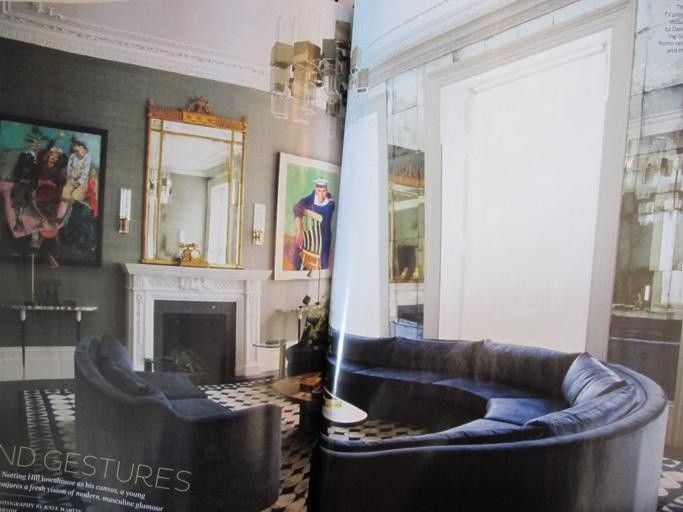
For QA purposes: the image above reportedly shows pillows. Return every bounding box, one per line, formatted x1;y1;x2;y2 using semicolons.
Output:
100;332;170;406
320;330;638;450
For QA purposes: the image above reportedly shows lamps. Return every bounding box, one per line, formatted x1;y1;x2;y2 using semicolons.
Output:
252;203;266;246
117;187;132;234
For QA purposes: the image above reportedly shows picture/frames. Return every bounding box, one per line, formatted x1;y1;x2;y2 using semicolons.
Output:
272;152;339;281
0;113;108;266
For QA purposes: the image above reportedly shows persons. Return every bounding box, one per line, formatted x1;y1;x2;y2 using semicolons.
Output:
9;139;97;236
283;176;334;271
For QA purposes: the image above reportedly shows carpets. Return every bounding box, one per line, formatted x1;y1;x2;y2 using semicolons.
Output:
18;371;683;512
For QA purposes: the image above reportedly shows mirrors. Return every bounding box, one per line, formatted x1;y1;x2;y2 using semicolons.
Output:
386;144;426;283
140;99;248;269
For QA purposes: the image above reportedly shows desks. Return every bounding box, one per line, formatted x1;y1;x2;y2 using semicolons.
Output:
277;302;324;344
273;372;319;440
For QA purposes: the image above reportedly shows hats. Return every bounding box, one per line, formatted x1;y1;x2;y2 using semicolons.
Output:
313;177;330;188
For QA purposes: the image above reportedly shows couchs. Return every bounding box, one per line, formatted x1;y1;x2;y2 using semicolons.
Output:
74;331;282;512
307;329;669;512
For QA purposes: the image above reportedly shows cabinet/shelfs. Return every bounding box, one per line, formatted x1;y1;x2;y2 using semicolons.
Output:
611;315;682;463
